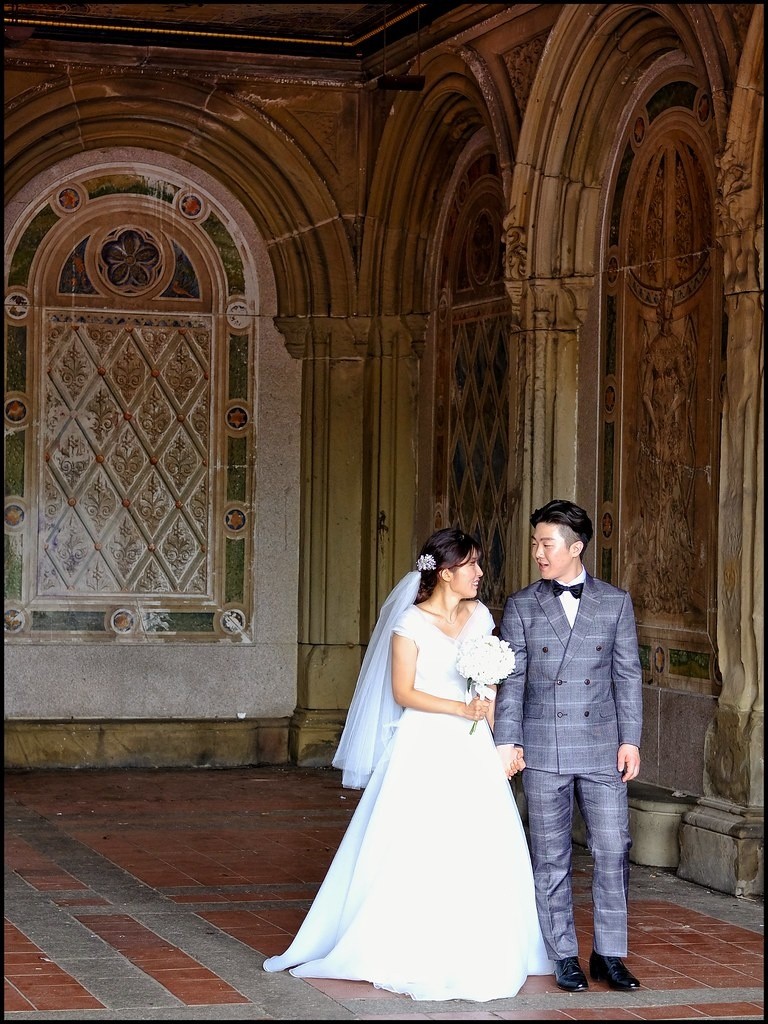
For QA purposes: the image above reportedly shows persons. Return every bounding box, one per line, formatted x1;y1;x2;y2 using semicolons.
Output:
264;528;554;1002
494;500;643;994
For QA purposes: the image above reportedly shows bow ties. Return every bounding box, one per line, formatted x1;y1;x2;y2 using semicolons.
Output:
551;579;584;599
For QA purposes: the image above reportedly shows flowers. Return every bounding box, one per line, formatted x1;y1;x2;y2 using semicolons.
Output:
454;631;515;735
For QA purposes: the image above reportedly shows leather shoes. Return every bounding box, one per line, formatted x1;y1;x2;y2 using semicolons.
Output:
590;951;639;991
556;957;588;992
